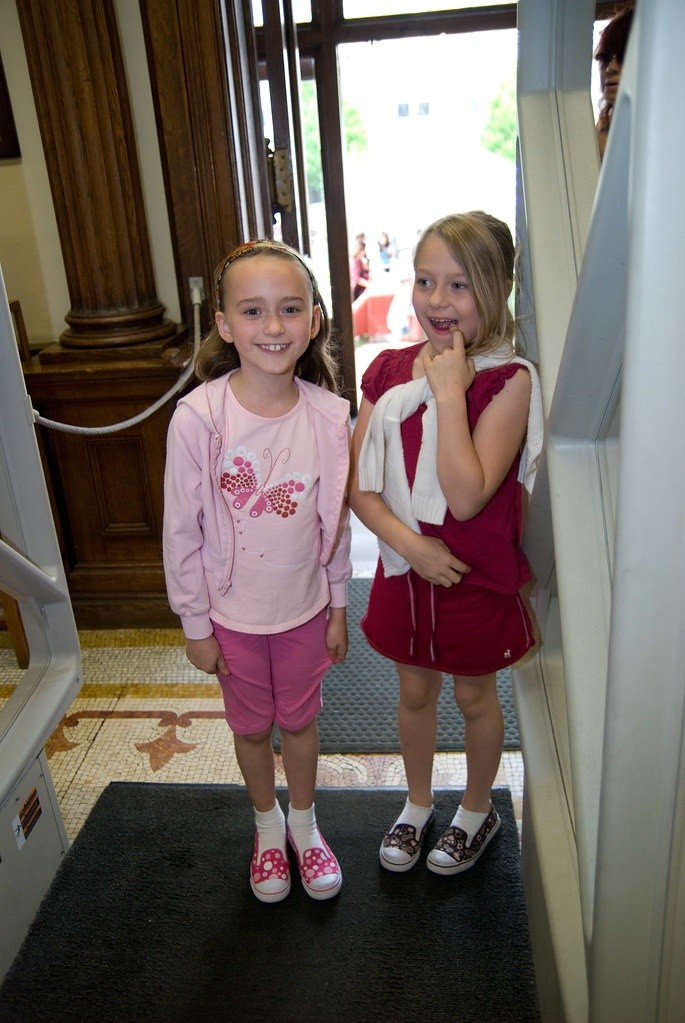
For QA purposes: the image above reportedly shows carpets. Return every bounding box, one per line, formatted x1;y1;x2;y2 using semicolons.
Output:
267;577;519;754
0;780;544;1023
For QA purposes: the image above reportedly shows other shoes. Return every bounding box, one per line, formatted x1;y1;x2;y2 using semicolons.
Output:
250;830;292;904
426;800;501;875
379;803;435;872
288;826;343;900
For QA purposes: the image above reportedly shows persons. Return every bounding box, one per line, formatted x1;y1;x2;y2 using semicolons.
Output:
348;211;545;877
353;230;423;341
162;239;353;902
596;8;634;160
